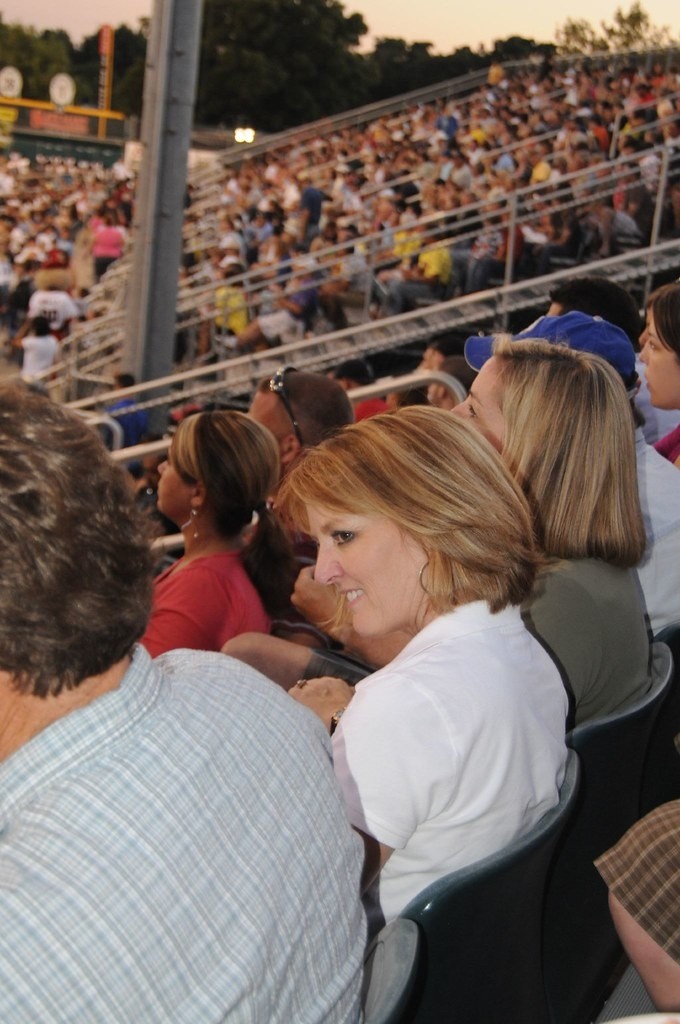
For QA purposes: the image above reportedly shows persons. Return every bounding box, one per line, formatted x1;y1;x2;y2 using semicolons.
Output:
0;43;680;728
595;800;679;1024
275;406;570;932
0;388;367;1024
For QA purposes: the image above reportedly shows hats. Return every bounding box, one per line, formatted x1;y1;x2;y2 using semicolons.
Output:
462;311;639;402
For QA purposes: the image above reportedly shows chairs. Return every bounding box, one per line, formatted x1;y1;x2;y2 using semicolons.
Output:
341;618;680;1024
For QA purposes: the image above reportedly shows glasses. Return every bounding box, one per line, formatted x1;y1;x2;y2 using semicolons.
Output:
269;365;306;447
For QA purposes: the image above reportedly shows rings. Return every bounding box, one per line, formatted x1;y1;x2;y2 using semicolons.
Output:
296;679;307;688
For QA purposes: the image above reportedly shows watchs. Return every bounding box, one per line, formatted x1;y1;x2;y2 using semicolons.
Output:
330;706;347;735
